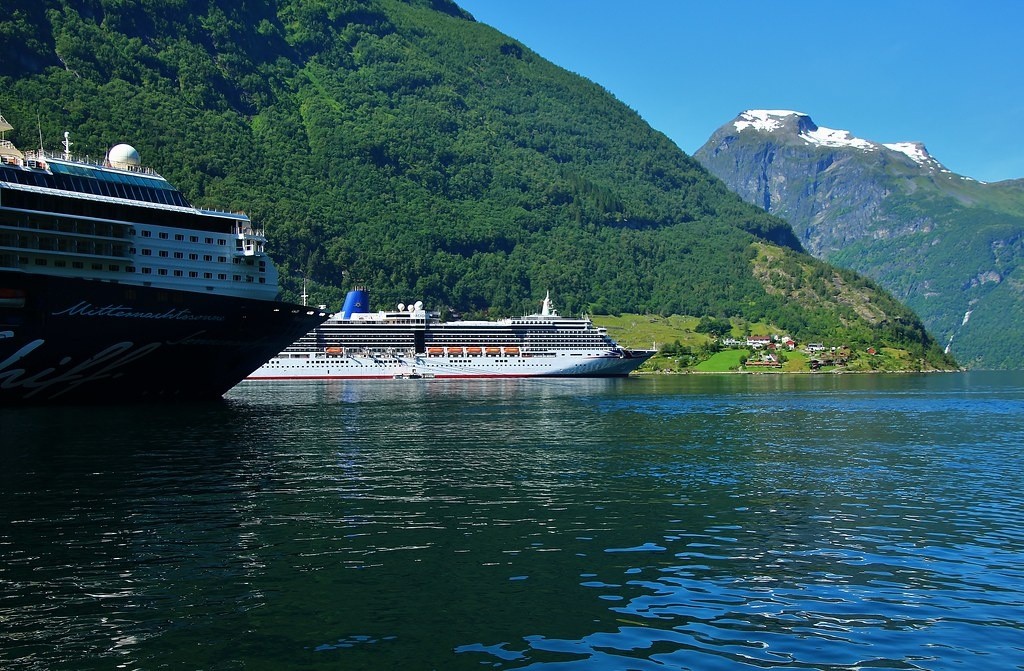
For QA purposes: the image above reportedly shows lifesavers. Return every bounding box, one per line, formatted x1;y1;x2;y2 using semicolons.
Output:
356;303;361;308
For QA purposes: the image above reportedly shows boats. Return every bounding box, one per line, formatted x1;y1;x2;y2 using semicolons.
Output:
466;348;482;357
326;348;343;357
0;108;337;411
504;347;519;356
447;348;464;356
428;348;444;356
486;348;501;356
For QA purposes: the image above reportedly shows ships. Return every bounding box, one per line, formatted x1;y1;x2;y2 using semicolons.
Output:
243;287;659;381
